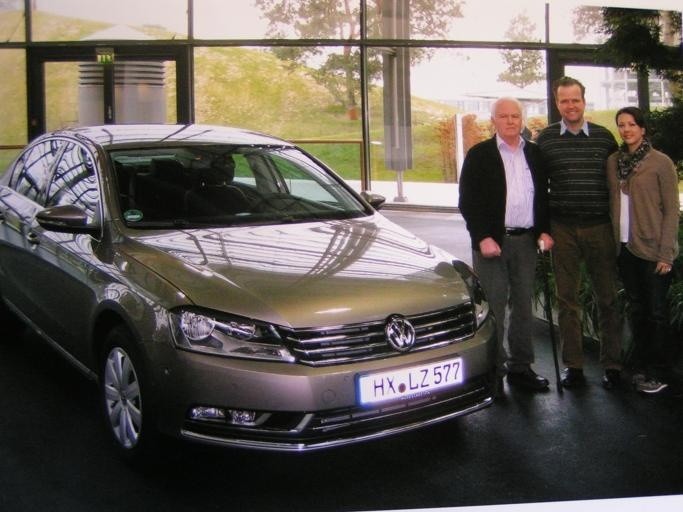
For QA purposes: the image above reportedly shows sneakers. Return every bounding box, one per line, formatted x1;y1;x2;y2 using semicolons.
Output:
632;374;668;394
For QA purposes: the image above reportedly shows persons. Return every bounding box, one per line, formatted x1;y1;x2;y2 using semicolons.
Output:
532;78;625;393
606;107;679;395
456;97;556;399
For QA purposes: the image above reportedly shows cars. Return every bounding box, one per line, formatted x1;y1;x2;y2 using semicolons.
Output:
0;124;496;459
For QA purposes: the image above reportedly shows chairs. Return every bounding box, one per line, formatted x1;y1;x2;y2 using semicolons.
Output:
115;151;252;217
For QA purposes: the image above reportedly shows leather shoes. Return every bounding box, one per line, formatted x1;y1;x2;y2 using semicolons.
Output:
507;368;549;387
560;367;583;386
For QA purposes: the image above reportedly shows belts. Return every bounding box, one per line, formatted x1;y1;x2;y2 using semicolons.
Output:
505;227;528;240
600;368;619;388
